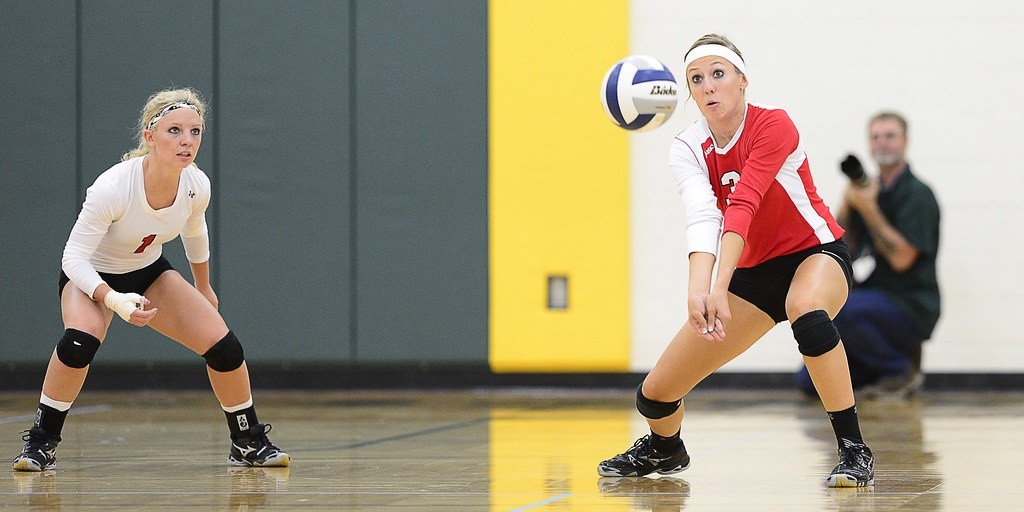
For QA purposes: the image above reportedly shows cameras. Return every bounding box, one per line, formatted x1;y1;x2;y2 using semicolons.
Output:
840;153;871;188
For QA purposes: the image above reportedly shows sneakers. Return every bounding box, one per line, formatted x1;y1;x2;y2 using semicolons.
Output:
597;432;691;478
826;437;875;487
13;420;62;472
227;423;290;469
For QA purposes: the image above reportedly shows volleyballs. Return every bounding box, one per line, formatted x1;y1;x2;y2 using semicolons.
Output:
602;54;680;134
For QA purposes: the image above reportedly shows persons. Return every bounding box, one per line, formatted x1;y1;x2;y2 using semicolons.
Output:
596;32;876;488
796;111;941;403
10;87;291;473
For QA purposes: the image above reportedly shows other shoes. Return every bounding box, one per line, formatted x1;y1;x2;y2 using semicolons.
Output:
862;368;926;401
